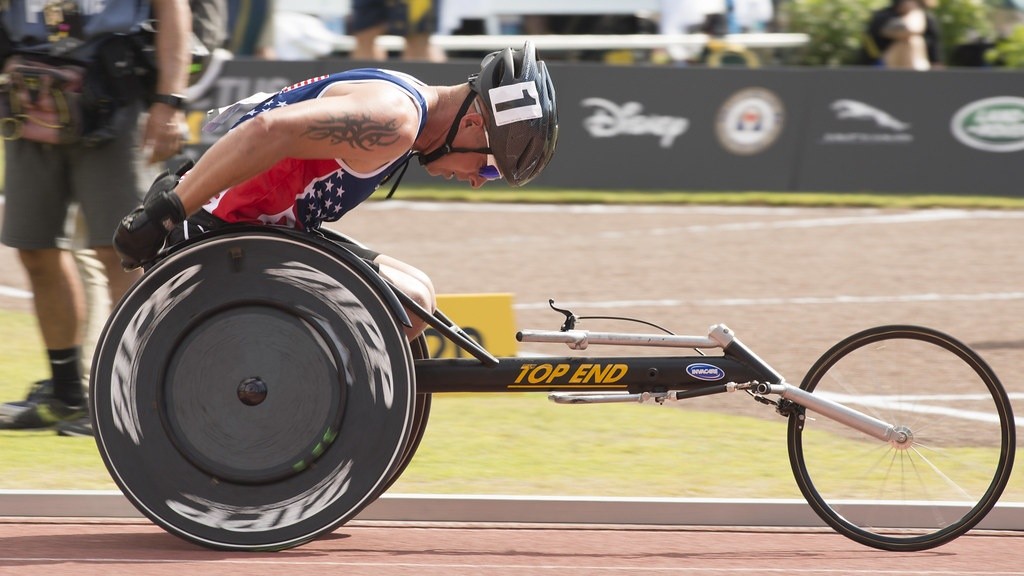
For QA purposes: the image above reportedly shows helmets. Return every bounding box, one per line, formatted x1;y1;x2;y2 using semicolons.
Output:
471;40;559;188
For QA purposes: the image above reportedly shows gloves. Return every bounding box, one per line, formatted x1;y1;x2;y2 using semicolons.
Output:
112;191;187;274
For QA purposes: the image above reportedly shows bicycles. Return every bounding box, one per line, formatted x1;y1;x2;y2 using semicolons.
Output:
89;221;1016;555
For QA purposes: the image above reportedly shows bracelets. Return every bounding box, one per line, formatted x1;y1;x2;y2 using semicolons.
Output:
154;94;192;110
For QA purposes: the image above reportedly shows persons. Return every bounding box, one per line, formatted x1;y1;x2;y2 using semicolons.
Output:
190;0;228;57
854;1;941;72
949;0;1024;68
686;14;762;68
603;17;672;65
114;42;558;343
343;1;447;63
1;0;192;436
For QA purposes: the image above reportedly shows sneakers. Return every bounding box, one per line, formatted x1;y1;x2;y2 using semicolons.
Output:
1;378;92;438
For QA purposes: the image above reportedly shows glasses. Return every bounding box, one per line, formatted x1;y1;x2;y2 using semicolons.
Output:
474;92;503;182
